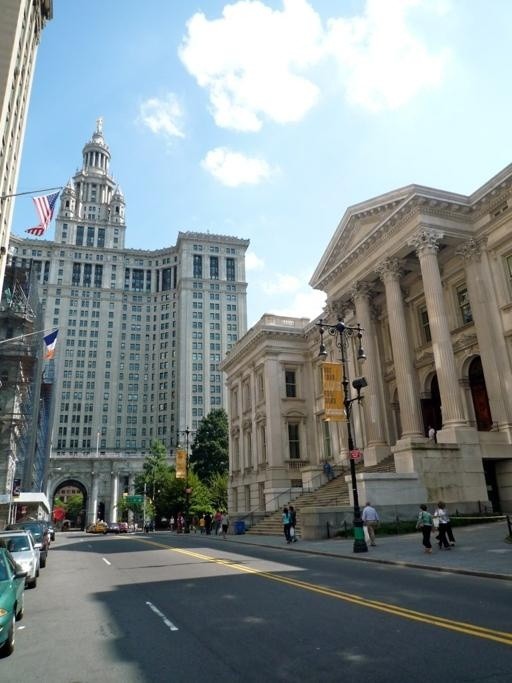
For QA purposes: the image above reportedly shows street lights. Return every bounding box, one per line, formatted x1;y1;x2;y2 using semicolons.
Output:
173;425;201;534
38;467;61;492
313;314;372;554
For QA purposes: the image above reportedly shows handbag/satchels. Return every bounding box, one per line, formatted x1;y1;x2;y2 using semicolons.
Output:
290;528;294;537
432;517;440;529
419;520;425;527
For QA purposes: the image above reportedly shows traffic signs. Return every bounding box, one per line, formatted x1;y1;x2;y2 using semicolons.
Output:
127;495;144;502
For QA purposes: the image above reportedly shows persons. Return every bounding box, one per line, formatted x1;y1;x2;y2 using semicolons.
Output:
280;507;293;544
287;505;297;542
144;520;153;537
433;500;451;550
323;459;337;481
168;508;223;536
435;505;456;547
219;510;229;539
361;501;380;546
415;503;435;553
427;424;436;440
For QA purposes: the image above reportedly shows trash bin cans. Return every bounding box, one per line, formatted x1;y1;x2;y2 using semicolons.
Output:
234;522;245;535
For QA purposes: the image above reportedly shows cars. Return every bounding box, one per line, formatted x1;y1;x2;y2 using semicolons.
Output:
0;517;56;655
87;518;130;534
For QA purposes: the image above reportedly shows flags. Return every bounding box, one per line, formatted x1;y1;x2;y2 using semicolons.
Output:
175;448;187;478
24;190;60;237
320;361;348;422
42;328;59;361
